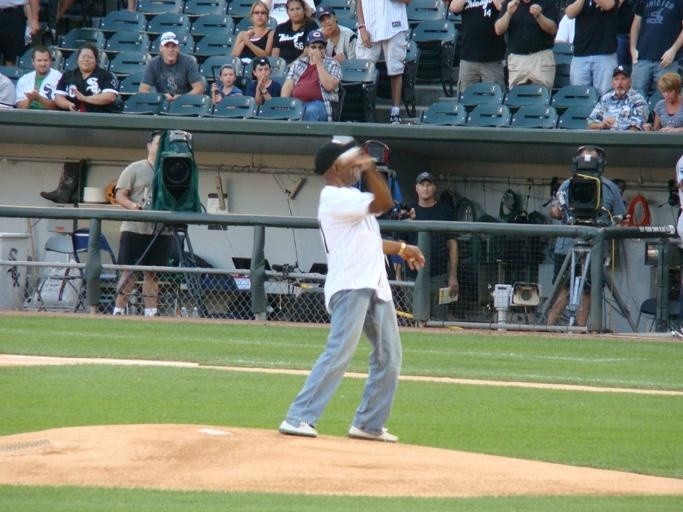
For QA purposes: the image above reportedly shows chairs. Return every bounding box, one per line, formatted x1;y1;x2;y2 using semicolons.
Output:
27;228;253;322
1;1;683;134
456;228;533;284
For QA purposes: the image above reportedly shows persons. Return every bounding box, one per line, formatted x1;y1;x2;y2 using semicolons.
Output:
278;133;402;443
112;133;166;315
545;145;631;327
673;155;683;336
392;172;462;322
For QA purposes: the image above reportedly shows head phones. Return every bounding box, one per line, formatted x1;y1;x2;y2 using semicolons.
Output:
574;145;610;175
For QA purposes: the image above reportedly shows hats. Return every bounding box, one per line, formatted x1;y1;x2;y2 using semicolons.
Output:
315;5;333;20
416;172;435;184
251;56;271;80
305;30;327;46
614;65;630;76
313;135;360;174
159;32;180;46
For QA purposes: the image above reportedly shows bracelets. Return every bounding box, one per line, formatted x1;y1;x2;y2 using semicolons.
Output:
398;243;406;257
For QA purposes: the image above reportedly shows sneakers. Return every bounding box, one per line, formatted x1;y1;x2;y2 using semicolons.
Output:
279;419;317;437
388;114;403;124
348;424;399;442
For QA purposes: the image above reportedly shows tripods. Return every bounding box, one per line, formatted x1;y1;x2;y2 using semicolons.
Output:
536;241;637;334
100;223;211;319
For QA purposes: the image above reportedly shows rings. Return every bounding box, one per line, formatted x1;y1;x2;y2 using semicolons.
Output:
410;258;416;262
